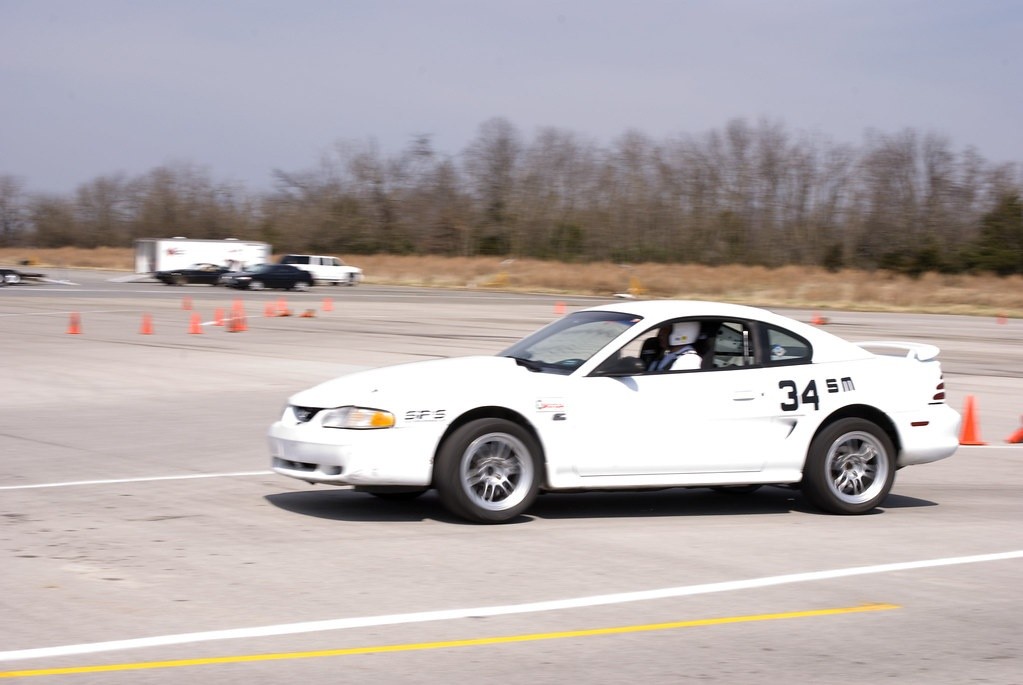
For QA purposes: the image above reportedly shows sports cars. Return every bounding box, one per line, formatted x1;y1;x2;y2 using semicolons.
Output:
270;298;964;522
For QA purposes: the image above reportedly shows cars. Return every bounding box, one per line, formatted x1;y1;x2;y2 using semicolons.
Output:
0;263;47;286
219;262;314;295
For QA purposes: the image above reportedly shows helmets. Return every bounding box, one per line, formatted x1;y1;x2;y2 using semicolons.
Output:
657;320;700;346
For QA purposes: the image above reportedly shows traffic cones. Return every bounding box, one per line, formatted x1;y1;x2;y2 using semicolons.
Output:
138;310;154;334
189;311;206;335
323;295;335;312
1007;412;1023;448
995;311;1006;329
182;294;195;311
211;305;225;327
806;310;828;326
66;309;83;333
295;308;316;319
225;290;249;332
267;298;291;318
555;301;566;316
958;394;988;447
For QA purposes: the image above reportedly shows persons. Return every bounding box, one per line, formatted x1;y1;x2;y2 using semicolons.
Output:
646;322;703;370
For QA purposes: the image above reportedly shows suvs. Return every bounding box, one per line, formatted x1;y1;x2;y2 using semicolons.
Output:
275;254;365;284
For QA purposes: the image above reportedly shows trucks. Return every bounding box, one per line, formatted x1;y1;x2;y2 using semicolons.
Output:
135;236;272;284
